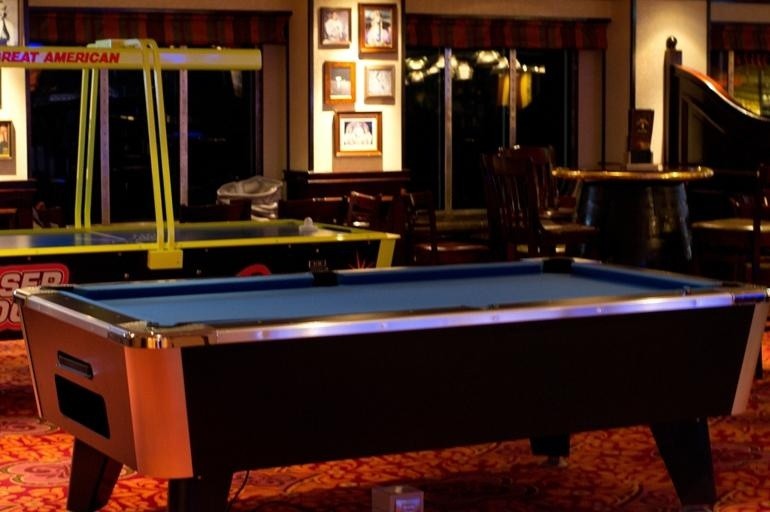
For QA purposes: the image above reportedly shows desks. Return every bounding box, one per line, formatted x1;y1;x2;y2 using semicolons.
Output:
9;255;770;511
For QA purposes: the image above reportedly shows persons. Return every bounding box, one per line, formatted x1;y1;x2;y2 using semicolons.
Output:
323;9;344;40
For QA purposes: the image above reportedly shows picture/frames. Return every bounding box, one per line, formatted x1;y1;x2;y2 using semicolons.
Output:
320;58;358;109
363;64;397;103
0;118;16;165
316;4;353;51
355;1;400;58
330;110;386;161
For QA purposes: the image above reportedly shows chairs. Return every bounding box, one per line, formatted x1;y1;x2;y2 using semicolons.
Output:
281;137;769;384
1;169;282;228
